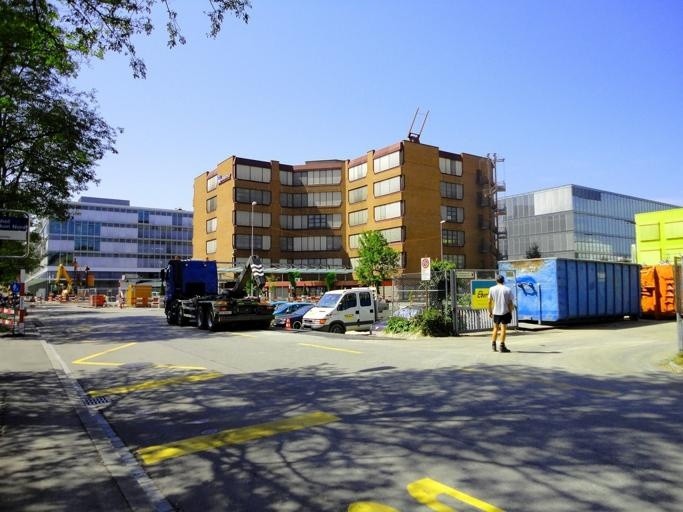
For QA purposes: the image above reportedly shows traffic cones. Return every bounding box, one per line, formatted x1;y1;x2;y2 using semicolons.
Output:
284;318;293;330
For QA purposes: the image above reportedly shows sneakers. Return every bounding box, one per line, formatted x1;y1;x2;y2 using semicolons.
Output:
492;344;510;352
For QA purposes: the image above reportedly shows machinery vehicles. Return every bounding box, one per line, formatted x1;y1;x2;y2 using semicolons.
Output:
160;255;274;330
50;265;75;298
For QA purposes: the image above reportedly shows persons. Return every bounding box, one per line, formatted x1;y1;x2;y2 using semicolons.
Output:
488;275;515;353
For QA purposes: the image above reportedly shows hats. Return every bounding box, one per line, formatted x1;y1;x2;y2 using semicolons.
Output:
496;275;504;280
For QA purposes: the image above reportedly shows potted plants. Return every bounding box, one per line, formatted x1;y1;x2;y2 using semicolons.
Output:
420;308;452;337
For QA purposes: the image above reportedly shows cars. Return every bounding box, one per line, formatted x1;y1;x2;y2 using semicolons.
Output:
269;300;314;329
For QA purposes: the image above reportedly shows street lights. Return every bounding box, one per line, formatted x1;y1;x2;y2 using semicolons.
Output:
440;220;446;260
251;201;257;297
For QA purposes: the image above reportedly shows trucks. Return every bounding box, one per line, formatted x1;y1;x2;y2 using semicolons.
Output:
303;287;393;334
